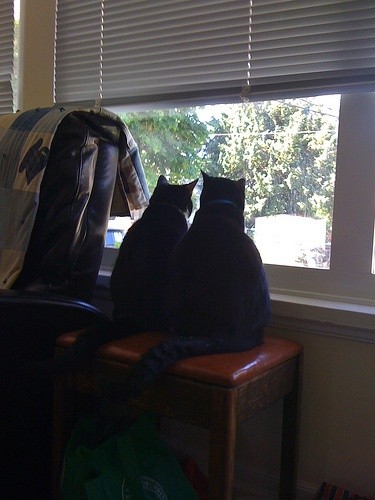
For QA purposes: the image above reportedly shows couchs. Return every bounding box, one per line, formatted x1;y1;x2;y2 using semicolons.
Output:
0;105;149;500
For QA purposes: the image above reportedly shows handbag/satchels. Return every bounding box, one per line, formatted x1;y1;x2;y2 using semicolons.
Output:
57;405;201;500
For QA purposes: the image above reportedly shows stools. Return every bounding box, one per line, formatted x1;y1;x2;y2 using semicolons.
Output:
51;328;303;500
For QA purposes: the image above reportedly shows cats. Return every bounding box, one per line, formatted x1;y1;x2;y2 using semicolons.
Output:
105;170;271;401
72;175;198;365
